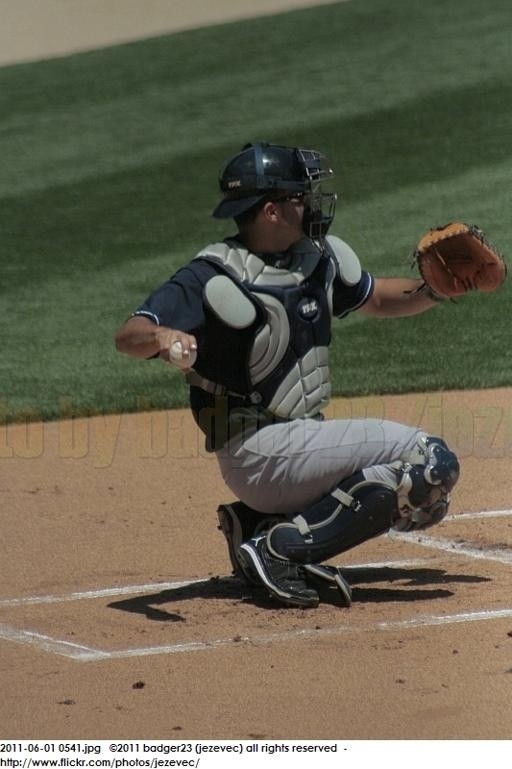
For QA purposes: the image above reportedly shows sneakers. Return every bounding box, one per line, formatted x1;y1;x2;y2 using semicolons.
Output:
216;503;319;608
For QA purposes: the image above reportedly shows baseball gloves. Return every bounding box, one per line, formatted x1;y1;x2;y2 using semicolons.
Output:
416;222;506;301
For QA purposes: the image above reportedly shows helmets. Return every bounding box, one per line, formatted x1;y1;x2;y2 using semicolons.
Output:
218;140;319;197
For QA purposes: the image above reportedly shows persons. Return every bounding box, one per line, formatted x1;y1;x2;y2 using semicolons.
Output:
116;141;459;608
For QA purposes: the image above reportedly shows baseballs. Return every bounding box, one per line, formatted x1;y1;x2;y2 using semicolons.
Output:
170;342;197;370
